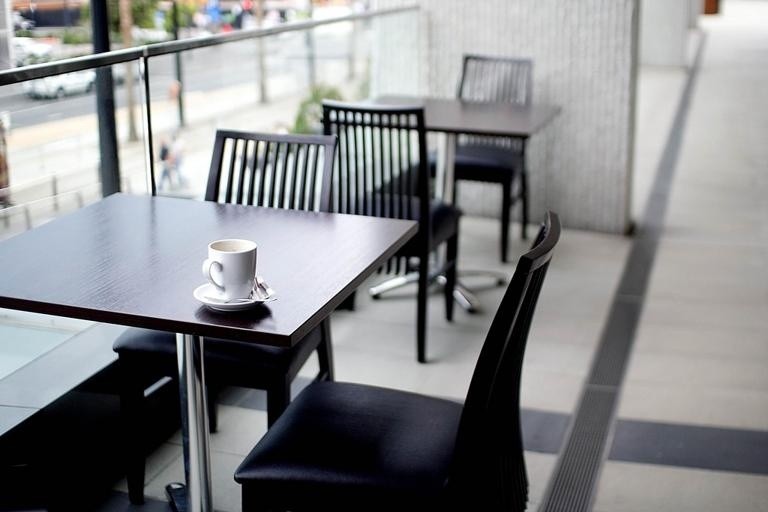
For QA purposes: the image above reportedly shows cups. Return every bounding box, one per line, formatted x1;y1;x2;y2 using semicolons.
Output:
202;238;258;298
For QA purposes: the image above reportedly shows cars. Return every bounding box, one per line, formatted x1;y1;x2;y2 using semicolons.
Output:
21;58;145;98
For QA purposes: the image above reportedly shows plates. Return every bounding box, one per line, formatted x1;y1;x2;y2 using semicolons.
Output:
193;281;272;311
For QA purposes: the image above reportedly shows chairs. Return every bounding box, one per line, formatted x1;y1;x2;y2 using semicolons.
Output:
0;54;562;510
